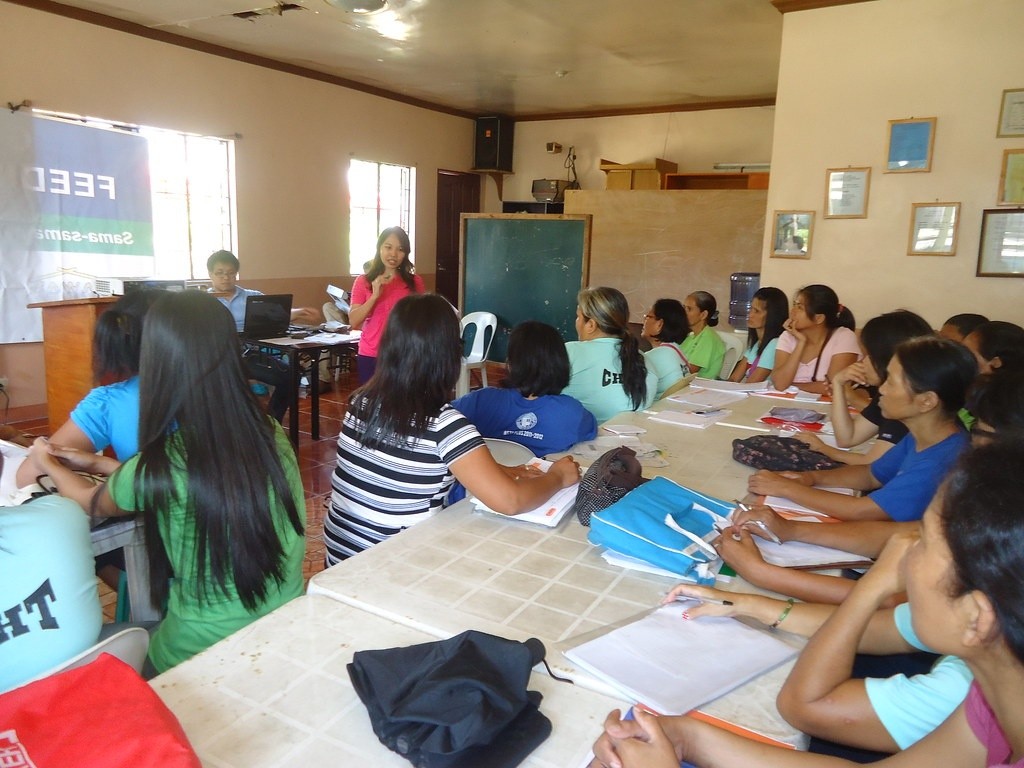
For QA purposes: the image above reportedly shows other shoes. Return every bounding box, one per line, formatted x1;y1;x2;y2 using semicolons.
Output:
318;380;332;394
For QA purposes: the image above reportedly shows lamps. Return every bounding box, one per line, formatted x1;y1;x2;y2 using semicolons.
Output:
546;142;562;154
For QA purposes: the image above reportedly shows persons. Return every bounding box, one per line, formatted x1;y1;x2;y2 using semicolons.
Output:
16;290;307;676
942;313;989;342
680;291;726;379
661;524;974;753
207;250;320;425
560;286;658;425
957;321;1024;431
711;383;1024;609
0;452;103;693
726;287;789;383
641;298;690;402
443;322;598;506
305;226;425;396
323;294;582;569
771;285;864;394
749;336;980;523
790;308;935;466
590;445;1024;768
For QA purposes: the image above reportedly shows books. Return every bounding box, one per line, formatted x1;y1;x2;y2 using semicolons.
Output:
759;391;861;414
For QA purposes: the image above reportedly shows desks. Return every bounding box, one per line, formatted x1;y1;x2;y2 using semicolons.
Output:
0;322;874;768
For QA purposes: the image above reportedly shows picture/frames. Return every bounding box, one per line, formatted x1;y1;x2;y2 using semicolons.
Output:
769;210;816;260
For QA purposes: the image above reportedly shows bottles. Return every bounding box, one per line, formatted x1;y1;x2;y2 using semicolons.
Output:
728;273;760;331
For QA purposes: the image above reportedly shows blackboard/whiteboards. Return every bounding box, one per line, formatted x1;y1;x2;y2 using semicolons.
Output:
458;213;594;365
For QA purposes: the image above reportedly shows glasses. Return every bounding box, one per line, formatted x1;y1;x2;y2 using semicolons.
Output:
213;271;237;278
683;304;700;312
643;314;658;320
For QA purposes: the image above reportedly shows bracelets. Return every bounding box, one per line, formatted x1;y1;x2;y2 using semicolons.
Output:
770;598;794;628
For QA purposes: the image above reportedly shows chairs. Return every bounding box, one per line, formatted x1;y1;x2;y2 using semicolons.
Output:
452;311;497;399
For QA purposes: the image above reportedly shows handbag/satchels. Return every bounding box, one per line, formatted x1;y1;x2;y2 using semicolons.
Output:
577;447;649;526
587;469;742;585
733;434;848;473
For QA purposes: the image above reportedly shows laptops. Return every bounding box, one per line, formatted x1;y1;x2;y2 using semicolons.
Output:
237;293;294;337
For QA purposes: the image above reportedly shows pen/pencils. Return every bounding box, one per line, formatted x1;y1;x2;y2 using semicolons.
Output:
662;592;732;607
825;374;832;383
734;498;781;545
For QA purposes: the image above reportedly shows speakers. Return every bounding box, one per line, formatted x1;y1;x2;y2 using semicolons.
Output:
475;115;515;171
